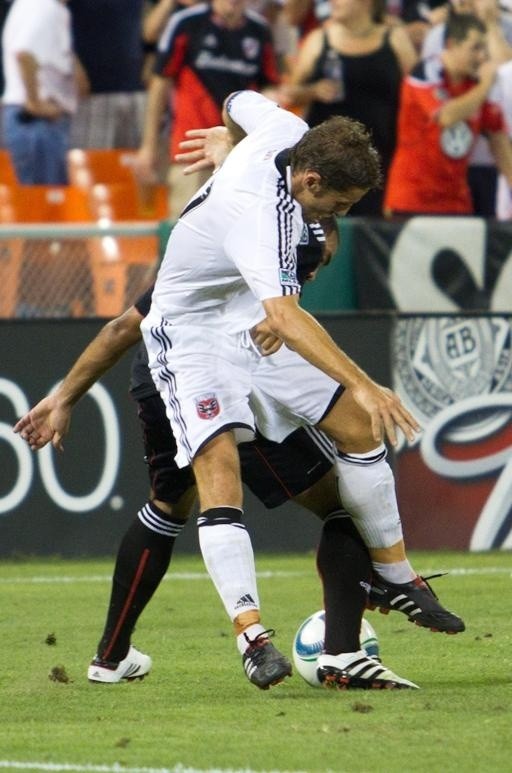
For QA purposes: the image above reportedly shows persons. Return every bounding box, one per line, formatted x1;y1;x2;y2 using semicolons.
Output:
0;0;93;186
140;89;468;690
11;216;429;691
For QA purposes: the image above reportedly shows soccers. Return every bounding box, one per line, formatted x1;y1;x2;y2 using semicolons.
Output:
292;609;379;688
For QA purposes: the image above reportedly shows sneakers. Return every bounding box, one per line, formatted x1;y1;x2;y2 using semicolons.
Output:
239;629;293;690
87;643;154;684
360;560;463;635
316;649;421;692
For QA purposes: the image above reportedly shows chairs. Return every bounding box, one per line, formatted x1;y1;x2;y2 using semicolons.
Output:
0;140;177;317
